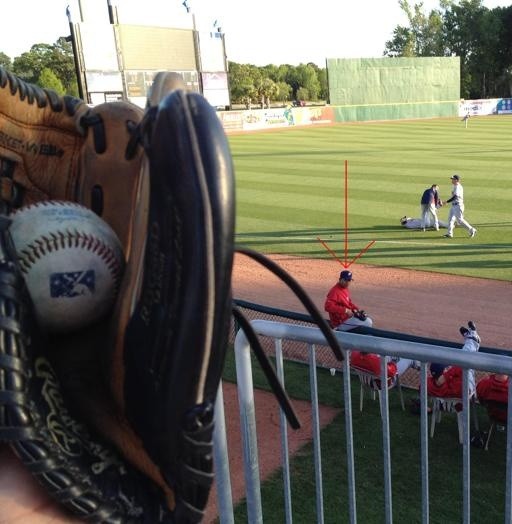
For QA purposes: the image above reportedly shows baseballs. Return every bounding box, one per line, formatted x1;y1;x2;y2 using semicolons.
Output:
7;201;126;329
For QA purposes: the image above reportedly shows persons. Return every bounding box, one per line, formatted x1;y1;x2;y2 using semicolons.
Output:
325;270;371;332
401;184;449;233
427;320;481;411
351;351;421;392
477;373;509;431
464;112;472;128
444;175;478;238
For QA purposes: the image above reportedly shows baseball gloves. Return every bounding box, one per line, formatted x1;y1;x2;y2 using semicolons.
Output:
353;310;367;320
0;65;235;523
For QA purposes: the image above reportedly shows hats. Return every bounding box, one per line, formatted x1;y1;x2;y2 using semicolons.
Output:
451;175;459;180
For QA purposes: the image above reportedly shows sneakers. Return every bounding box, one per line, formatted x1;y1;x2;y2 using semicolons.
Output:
460;321;481;343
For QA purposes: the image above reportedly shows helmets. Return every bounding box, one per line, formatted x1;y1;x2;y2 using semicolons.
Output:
340;271;352;280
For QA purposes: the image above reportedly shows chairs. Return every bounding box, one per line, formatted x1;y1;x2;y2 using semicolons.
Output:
352;364;406;415
430;391;508;451
